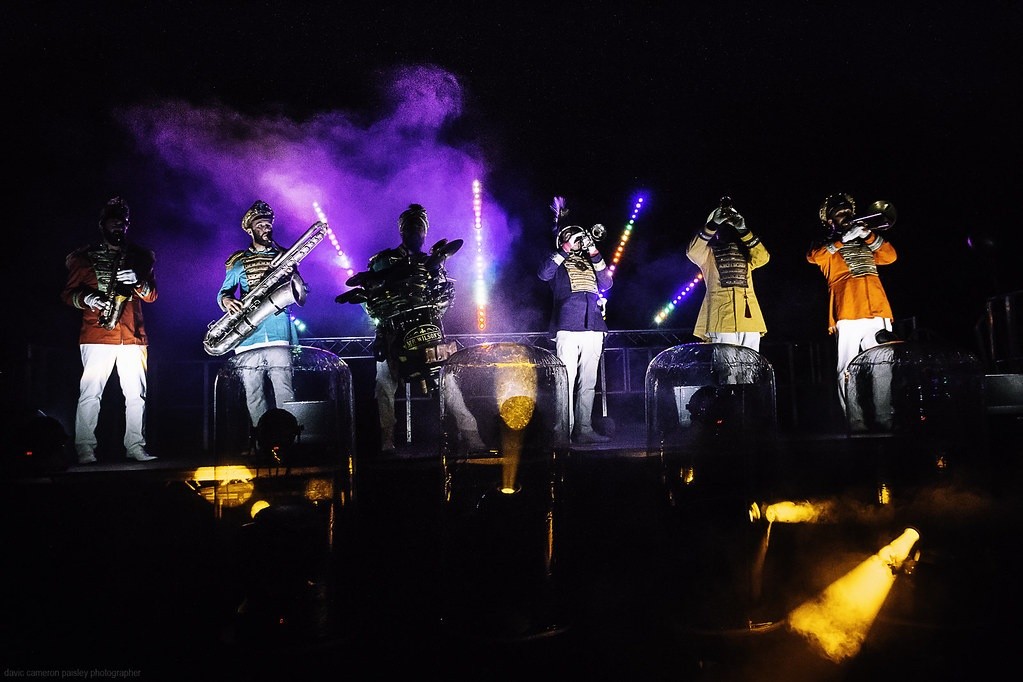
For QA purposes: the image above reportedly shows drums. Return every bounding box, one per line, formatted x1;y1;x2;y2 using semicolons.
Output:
382;306;451;383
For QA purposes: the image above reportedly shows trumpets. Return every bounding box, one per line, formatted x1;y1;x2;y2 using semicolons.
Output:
720;195;733;216
576;223;608;250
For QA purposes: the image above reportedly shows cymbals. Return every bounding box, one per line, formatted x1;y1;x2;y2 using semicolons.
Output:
334;237;465;319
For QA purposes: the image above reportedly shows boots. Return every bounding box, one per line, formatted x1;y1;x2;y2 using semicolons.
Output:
462;429;487;450
381;427;396;453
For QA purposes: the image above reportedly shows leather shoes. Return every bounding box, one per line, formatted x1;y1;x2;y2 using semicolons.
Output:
126;446;159;461
78;444;97;464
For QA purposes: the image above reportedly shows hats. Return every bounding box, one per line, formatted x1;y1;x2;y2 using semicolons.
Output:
706;206;737;224
819;193;856;226
549;197;586;256
99;196;129;225
397;203;429;237
241;200;274;231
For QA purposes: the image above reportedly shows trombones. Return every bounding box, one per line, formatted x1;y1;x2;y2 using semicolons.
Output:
842;199;898;232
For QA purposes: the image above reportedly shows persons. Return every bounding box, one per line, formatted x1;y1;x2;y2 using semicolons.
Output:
538;197;613;446
686;197;771;385
367;205;485;454
59;199;159;465
806;193;901;434
217;199;305;429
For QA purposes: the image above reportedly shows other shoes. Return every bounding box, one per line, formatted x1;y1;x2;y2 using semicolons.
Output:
884;421;903;432
851;421;868;433
579;432;612;444
555;431;569;449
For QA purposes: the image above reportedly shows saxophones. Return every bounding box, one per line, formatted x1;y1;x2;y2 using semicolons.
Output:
97;230;132;331
203;221;330;356
